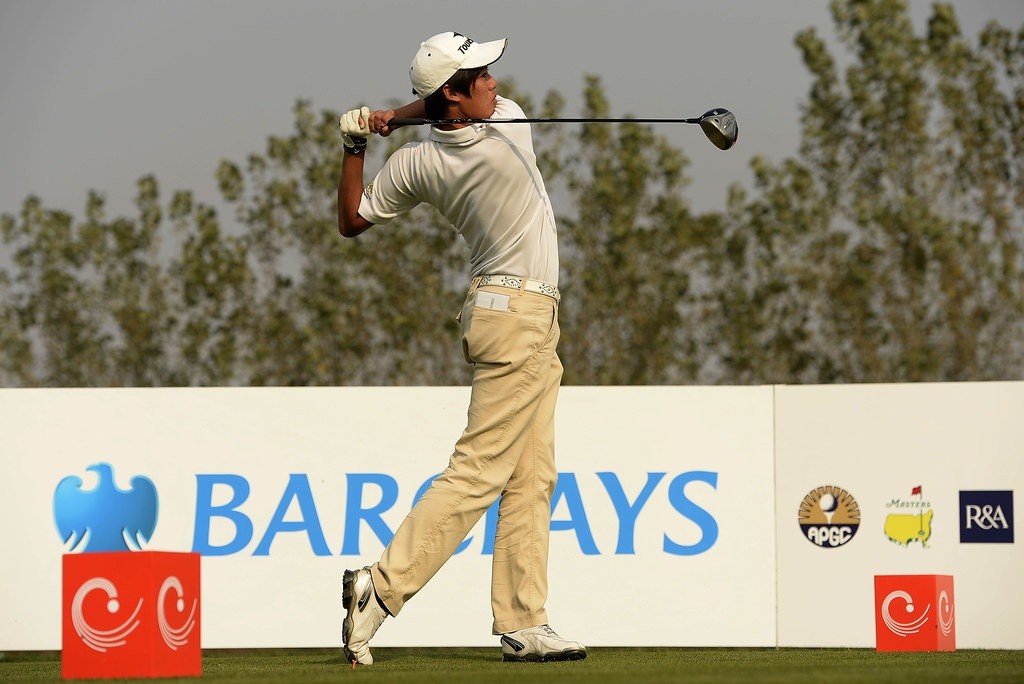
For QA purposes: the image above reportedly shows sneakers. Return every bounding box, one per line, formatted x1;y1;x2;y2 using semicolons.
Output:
342;567;389;666
500;625;588;662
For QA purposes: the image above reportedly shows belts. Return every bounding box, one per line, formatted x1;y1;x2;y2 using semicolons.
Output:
477;275;560;303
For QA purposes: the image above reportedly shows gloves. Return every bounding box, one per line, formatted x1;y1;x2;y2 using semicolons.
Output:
338;106;373;153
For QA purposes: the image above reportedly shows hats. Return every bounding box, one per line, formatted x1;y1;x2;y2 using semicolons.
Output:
408;31;508;99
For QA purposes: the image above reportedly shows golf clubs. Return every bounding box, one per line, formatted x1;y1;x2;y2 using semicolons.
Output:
387;107;739;150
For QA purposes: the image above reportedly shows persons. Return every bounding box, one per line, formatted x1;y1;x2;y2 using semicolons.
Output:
337;32;587;664
